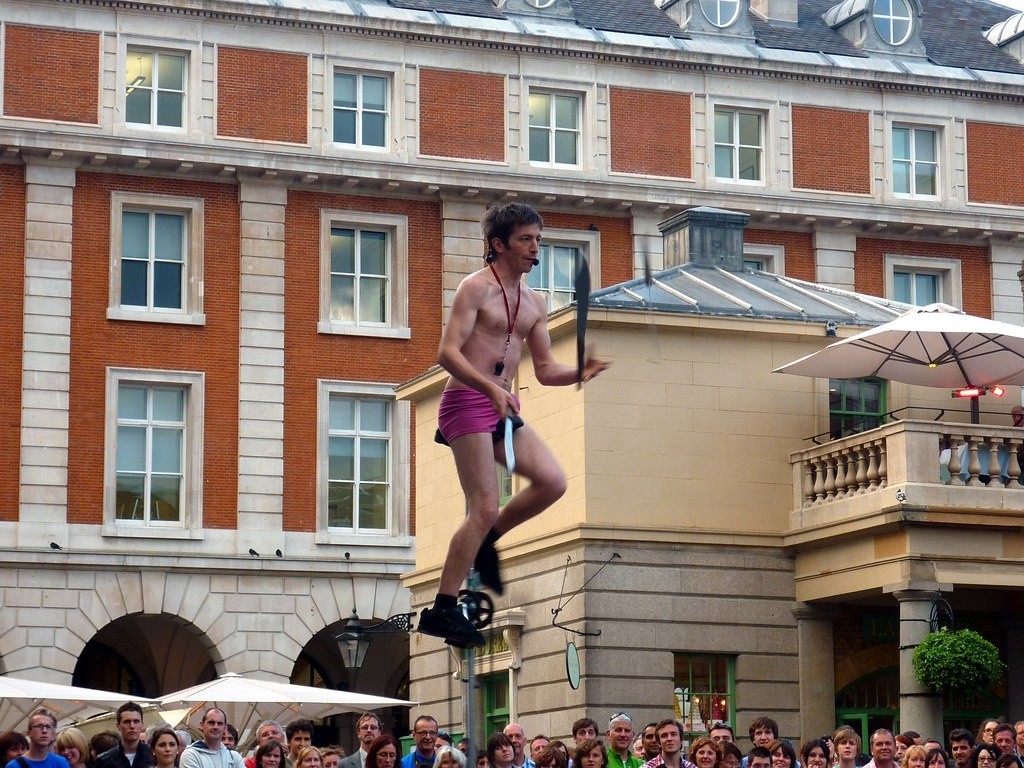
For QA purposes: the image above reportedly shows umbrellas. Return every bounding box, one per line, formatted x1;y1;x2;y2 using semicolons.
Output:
772;302;1024;388
0;672;419;757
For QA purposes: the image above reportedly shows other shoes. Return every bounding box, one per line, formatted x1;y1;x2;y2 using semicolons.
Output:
418;608;486;647
473;539;507;596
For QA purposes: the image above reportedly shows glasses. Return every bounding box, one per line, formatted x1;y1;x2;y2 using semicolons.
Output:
978;755;996;763
415;730;439;738
32;724;55;730
377;752;397;758
725;761;741;767
359;724;380;731
610;712;632;721
983;728;993;733
752;763;772;768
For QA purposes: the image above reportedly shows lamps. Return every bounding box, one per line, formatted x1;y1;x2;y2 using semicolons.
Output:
331;603;417;671
126;56;146;96
950;386;986;400
987;384;1006;398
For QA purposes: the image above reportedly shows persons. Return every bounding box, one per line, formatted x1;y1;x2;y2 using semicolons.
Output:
415;203;610;649
179;708;401;768
529;712;697;768
401;715;535;768
689;717;871;768
863;719;1024;768
1011;406;1024;485
0;703;190;768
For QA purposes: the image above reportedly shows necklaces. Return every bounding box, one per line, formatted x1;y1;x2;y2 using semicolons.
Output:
489;263;520;375
